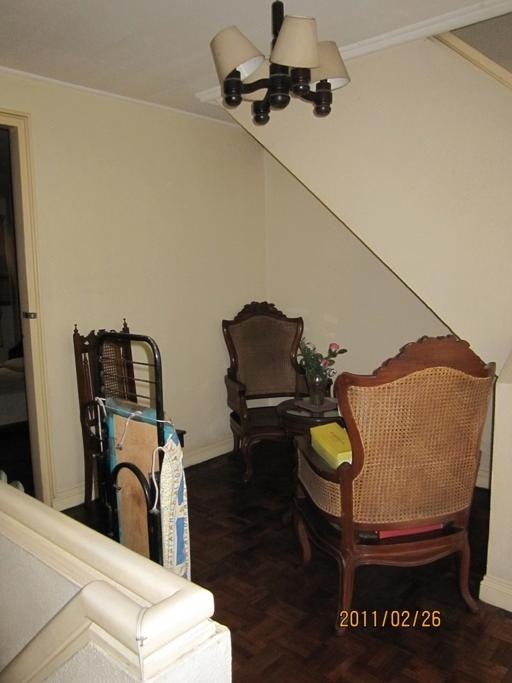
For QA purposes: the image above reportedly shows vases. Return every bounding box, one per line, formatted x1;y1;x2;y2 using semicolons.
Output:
308;372;328;407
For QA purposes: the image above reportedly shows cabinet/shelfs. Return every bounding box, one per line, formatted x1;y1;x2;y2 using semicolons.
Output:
89;326;192;582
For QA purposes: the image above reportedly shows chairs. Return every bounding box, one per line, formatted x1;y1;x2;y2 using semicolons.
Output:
73;317;187;517
291;332;499;635
221;300;333;483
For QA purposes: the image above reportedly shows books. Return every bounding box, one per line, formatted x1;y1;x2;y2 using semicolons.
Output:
374;523;447;539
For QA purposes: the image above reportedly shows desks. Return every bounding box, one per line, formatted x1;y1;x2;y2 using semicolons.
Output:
276;397;345;497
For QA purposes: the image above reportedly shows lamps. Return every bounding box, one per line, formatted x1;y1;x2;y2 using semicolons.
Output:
201;1;351;125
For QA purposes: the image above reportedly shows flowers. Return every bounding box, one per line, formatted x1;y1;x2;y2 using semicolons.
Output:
288;334;347;380
